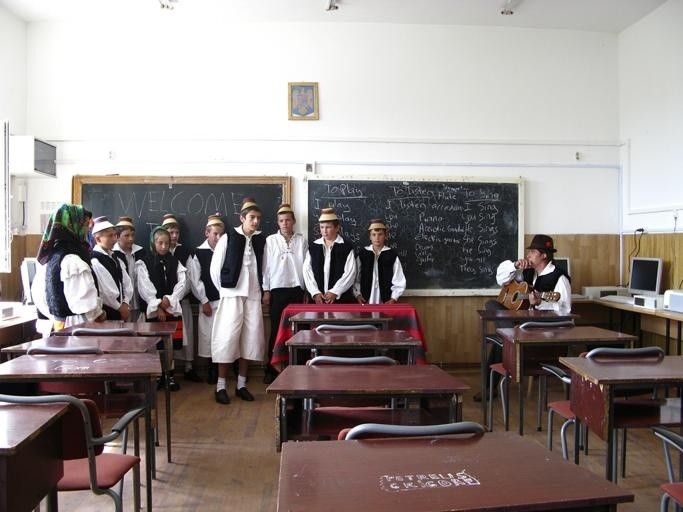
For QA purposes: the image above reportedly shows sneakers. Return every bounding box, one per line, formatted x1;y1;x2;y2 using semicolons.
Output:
473;387;497;401
207;365;218;384
156;368;180;392
263;368;276;384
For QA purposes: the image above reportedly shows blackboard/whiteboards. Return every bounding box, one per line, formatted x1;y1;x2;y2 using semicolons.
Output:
72;173;291;314
304;174;525;297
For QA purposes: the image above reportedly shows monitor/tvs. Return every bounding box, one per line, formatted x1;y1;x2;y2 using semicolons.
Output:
628;256;663;295
554;256;570;273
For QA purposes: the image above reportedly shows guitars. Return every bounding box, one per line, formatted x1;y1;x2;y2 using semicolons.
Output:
497;279;561;310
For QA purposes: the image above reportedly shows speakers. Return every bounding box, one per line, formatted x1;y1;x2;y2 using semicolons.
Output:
9;134;57;180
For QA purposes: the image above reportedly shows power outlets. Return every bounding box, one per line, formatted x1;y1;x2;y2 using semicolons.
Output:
639;227;649;233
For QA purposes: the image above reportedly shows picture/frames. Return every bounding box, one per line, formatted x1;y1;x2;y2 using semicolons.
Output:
287;81;320;121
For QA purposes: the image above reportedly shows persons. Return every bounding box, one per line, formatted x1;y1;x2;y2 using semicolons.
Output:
473;235;571;402
261;204;308;382
303;209;357;305
352;218;406;304
189;211;226;384
209;197;265;404
160;213;198;381
136;225;188;392
32;204;146;336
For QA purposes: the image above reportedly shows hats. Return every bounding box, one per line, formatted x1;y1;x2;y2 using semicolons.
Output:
114;217;136;229
318;208;339;222
277;203;294;214
240;197;258;211
207;212;225;229
526;235;557;253
91;215;114;235
162;214;178;227
367;219;387;231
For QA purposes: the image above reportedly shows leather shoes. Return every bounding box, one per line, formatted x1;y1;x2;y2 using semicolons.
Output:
183;369;204;383
235;386;254;401
214;387;230;404
